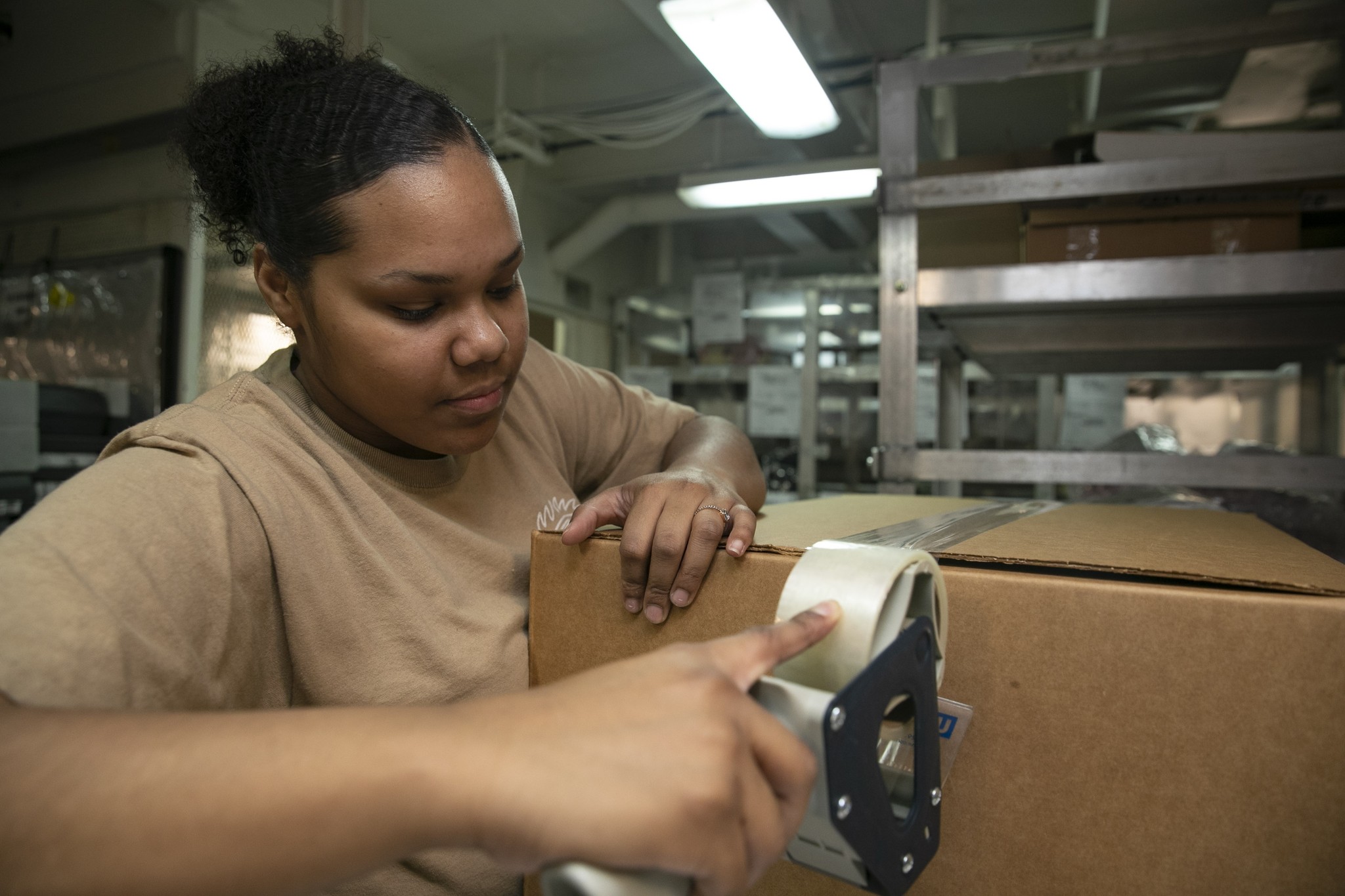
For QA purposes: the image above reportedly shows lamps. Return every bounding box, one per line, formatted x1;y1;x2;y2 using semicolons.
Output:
676;156;884;211
659;1;840;140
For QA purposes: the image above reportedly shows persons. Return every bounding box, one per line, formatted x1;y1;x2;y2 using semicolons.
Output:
0;23;846;896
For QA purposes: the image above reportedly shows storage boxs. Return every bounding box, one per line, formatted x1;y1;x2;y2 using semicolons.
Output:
527;492;1345;896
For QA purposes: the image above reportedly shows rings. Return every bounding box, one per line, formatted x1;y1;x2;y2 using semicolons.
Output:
694;505;731;528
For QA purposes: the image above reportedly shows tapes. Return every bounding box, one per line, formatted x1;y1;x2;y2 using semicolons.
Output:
772;540;952;740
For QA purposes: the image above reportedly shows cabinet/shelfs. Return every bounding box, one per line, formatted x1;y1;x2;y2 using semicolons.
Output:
874;27;1345;495
